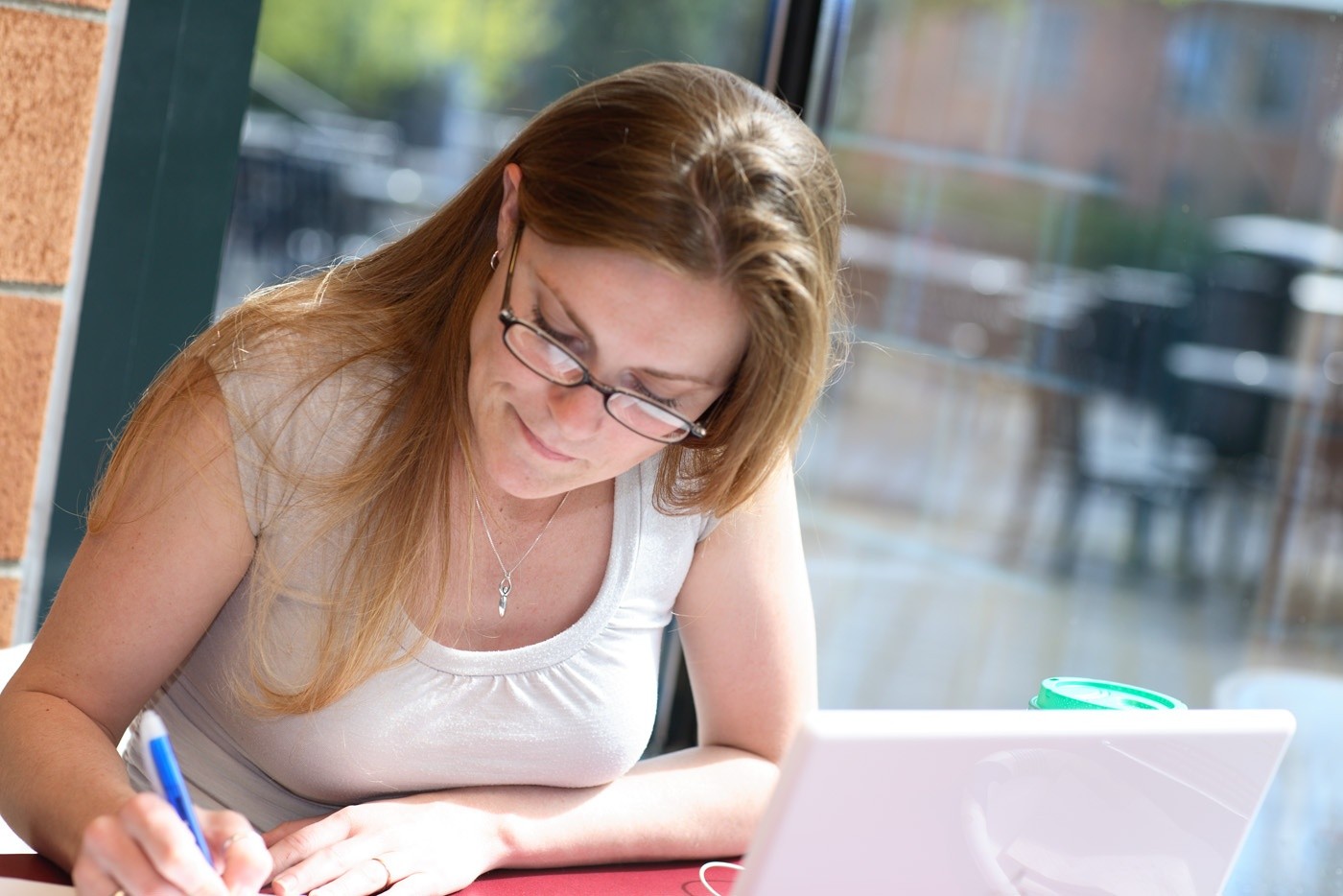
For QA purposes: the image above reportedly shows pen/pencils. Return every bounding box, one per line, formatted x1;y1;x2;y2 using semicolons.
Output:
139;710;212;864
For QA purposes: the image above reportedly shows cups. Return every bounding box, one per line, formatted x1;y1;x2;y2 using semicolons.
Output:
1027;676;1188;710
1214;667;1343;896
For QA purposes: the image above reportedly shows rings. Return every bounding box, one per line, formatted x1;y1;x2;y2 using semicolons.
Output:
113;889;125;896
220;830;265;858
373;858;391;891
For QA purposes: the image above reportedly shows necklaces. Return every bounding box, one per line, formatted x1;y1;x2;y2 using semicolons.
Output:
473;487;571;618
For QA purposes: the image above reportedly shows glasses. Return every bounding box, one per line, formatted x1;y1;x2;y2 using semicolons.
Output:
497;218;726;444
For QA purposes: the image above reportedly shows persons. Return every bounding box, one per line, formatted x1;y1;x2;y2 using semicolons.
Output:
0;64;847;896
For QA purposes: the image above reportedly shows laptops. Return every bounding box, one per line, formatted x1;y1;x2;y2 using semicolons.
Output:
727;705;1298;896
